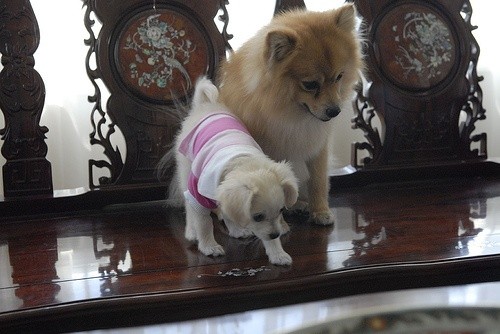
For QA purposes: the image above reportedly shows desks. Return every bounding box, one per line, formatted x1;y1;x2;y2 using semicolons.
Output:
0;160;500;334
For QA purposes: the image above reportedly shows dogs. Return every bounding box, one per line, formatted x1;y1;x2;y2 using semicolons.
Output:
174;76;300;266
216;3;364;226
